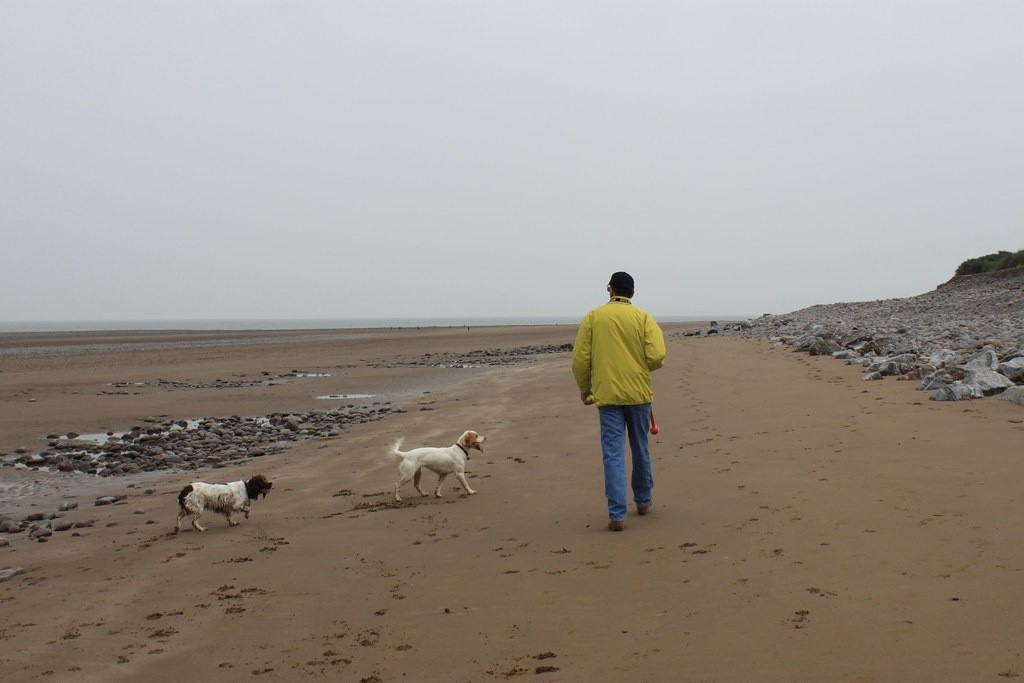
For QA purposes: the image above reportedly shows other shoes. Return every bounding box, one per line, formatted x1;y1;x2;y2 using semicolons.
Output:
608;519;625;530
636;500;652;515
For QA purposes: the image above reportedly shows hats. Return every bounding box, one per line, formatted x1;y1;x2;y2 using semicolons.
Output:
606;272;634;292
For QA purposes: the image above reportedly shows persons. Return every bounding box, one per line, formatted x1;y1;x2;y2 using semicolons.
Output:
572;271;664;529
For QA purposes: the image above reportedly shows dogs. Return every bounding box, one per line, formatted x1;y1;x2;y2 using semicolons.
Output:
385;429;487;504
173;474;274;534
